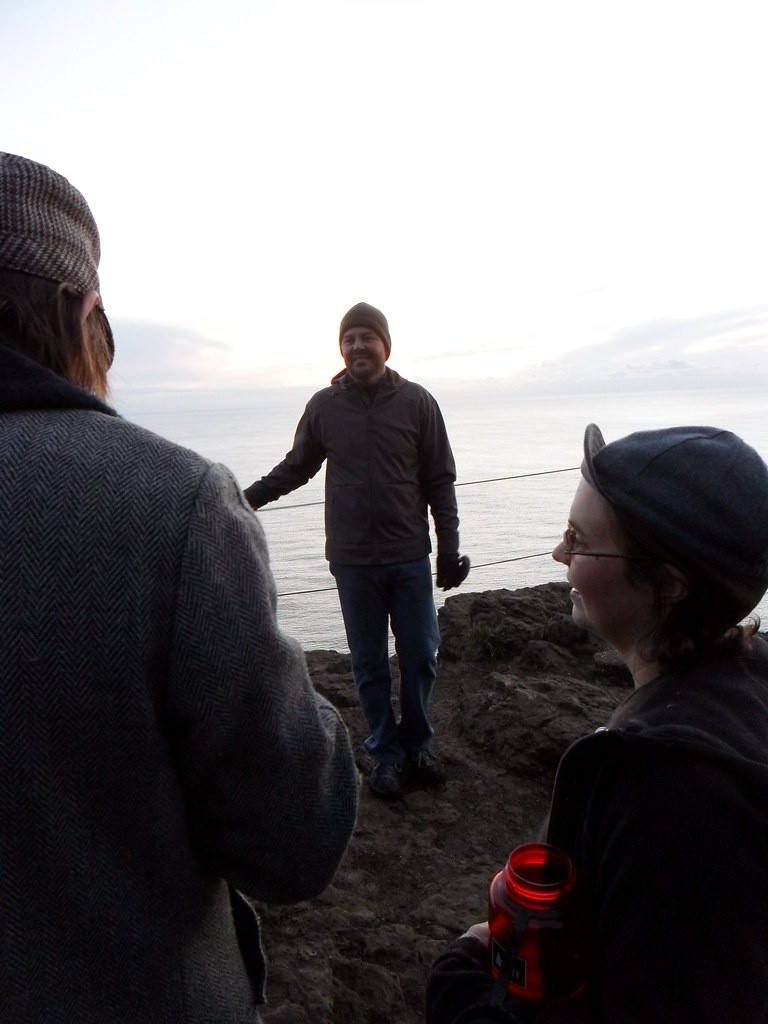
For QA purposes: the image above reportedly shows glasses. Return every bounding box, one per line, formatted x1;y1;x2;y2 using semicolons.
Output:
564;529;626;565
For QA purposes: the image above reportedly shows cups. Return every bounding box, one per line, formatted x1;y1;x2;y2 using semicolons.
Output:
489;841;586;1002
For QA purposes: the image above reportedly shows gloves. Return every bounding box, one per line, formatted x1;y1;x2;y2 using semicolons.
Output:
436;554;463;592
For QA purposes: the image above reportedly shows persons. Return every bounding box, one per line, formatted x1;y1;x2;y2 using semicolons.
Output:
0;152;368;1024
423;418;767;1023
244;302;464;803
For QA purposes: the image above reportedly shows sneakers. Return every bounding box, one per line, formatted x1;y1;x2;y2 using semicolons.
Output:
373;761;403;799
401;742;446;784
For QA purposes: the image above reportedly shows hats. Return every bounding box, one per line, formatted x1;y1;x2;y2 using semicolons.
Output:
0;152;115;369
581;423;768;581
339;302;391;362
